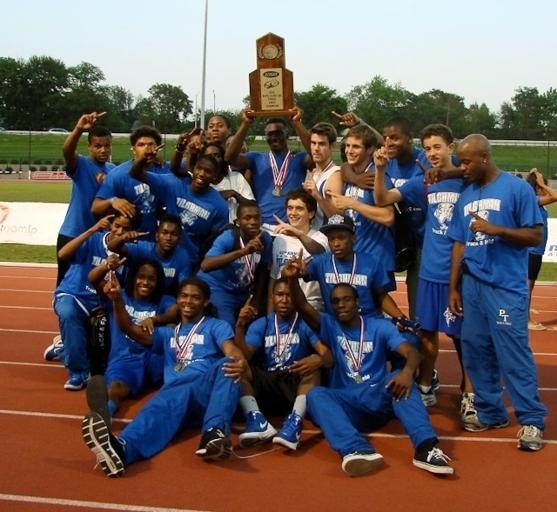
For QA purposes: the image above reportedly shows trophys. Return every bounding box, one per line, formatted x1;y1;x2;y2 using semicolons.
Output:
245;33;297;117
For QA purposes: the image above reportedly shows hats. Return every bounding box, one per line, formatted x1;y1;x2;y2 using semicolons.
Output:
319;214;353;234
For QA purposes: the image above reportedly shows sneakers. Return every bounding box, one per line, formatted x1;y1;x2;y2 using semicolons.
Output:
461;392;509;432
413;447;453;474
44;335;126;477
195;411;302;463
419;368;439;406
341;451;384;475
517;425;544;451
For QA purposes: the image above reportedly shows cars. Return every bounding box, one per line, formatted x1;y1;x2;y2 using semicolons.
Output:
0;125;5;130
48;127;68;132
